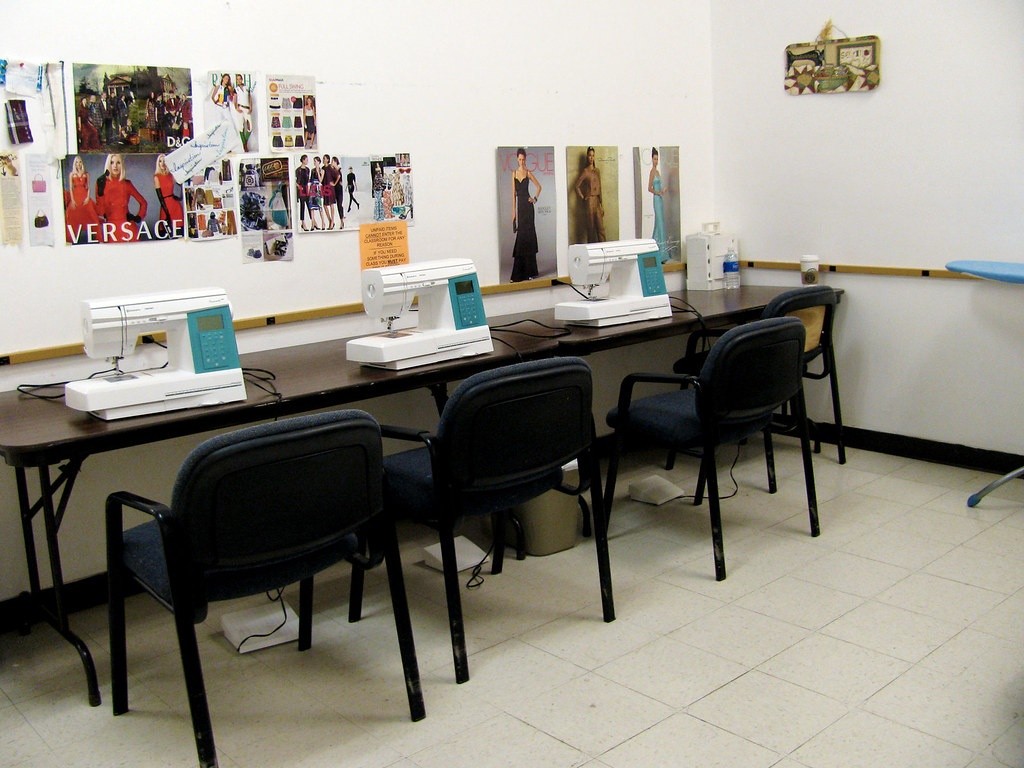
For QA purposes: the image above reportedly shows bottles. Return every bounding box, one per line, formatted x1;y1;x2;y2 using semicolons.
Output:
723;247;741;289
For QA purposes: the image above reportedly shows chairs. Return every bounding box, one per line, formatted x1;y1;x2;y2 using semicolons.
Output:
349;356;617;685
603;317;821;581
666;285;847;495
105;409;426;768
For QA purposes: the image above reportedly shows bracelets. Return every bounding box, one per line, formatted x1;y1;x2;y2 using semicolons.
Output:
534;197;537;204
583;195;586;199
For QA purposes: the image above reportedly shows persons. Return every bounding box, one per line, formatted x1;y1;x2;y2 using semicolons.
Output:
576;146;606;244
509;148;541;283
374;154;409;220
648;147;670;264
64;71;360;243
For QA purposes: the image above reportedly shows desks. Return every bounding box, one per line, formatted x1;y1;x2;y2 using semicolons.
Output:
0;285;803;707
946;259;1024;508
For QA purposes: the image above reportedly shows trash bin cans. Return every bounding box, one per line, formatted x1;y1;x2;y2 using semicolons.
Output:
501;458;586;558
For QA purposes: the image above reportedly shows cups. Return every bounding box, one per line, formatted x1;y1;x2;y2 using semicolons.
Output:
703;222;720;233
801;254;820;287
